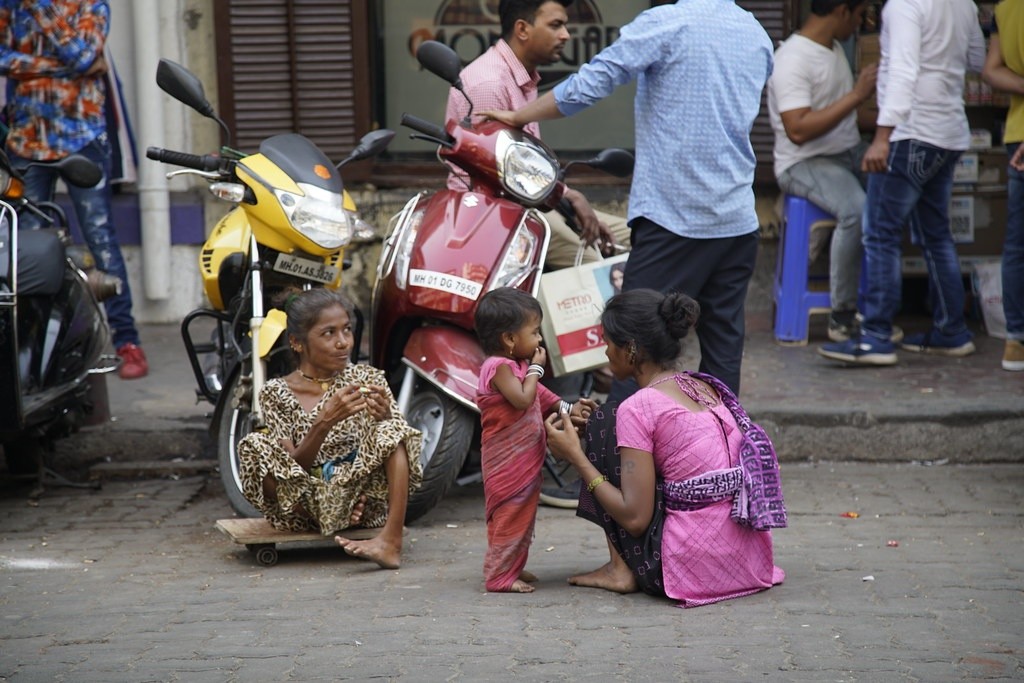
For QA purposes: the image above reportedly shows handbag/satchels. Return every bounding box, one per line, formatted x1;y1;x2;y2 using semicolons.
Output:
538;238;631;377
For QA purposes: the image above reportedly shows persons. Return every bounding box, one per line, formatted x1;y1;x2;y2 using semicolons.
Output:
472;286;592;593
765;0;905;346
981;0;1024;370
237;287;423;570
816;0;986;364
472;0;775;403
0;0;150;380
544;288;789;609
437;0;632;272
610;262;628;296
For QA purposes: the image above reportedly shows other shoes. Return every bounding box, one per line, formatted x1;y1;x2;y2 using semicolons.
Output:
1002;339;1024;371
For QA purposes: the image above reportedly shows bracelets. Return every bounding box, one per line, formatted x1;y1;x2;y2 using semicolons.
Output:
586;475;608;492
559;400;573;415
525;363;544;378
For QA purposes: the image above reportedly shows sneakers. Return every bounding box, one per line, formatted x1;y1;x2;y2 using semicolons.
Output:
828;313;904;343
818;332;898;366
901;327;975;356
116;344;146;378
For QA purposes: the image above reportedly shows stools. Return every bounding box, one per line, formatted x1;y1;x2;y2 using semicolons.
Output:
768;193;840;348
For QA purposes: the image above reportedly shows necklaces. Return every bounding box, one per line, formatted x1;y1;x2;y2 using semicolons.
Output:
297;367;339;390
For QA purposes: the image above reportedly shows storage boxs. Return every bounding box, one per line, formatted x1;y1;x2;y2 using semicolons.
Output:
854;34;1009;277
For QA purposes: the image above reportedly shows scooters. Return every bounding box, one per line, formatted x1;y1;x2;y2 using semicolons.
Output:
146;57;396;518
369;41;634;527
0;150;125;500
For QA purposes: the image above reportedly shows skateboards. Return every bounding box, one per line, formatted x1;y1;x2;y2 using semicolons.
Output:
215;507;404;566
